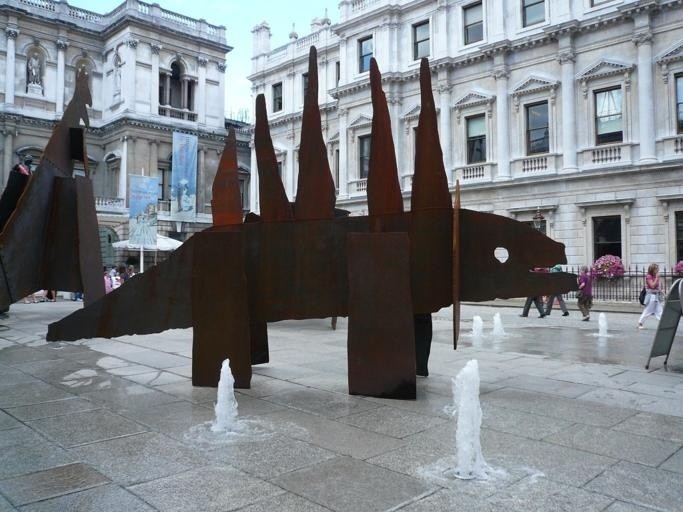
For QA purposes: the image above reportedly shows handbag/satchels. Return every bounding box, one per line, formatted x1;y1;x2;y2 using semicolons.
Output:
639;275;648;305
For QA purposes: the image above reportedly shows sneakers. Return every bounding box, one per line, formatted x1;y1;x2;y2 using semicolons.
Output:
562;311;569;316
24;299;56;304
582;315;590;321
538;310;550;318
519;314;528;317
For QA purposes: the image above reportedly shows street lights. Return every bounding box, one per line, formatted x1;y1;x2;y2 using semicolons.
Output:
20;151;33;175
530;208;545;233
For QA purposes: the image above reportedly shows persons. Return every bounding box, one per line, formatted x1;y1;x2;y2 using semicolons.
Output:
576;266;592;321
544;263;571;317
520;268;546;319
24;262;140;304
636;263;664;330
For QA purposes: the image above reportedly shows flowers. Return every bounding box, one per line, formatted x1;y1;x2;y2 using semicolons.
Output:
674;260;682;273
591;252;626;280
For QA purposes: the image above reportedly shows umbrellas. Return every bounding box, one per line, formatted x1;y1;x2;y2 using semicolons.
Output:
110;234;183;273
